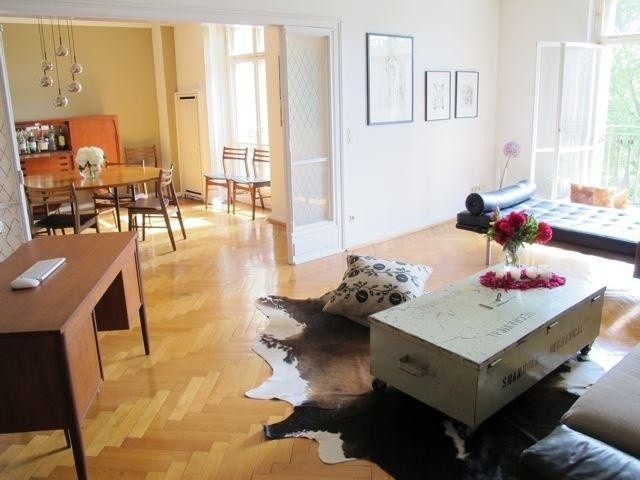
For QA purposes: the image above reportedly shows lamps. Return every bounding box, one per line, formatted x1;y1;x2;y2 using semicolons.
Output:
33;16;84;109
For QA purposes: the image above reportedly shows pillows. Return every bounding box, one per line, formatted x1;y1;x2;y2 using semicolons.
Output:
615;188;630;208
320;284;432;329
568;182;617;208
320;250;434;320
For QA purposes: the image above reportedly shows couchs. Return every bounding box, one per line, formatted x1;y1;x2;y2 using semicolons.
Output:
454;178;640;280
516;340;640;479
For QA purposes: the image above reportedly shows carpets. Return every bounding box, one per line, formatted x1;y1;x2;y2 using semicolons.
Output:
243;286;609;480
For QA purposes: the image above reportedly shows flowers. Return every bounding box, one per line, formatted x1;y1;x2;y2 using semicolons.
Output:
499;140;521;192
487;204;554;264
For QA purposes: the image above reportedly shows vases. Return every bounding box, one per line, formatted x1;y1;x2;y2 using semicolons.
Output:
504;244;521;271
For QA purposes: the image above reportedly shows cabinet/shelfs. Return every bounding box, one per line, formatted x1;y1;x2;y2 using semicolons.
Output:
15;120;75;202
70;115;122;198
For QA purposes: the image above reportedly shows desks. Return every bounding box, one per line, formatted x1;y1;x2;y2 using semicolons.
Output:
0;231;155;480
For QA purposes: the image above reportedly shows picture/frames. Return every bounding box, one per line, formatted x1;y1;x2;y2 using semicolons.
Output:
365;33;414;124
455;70;480;119
425;70;452;121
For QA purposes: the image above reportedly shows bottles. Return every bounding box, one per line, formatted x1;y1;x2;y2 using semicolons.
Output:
18;125;66;155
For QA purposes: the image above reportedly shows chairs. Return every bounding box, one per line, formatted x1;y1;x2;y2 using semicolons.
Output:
203;145;250;214
19;143;187;252
233;147;271;221
